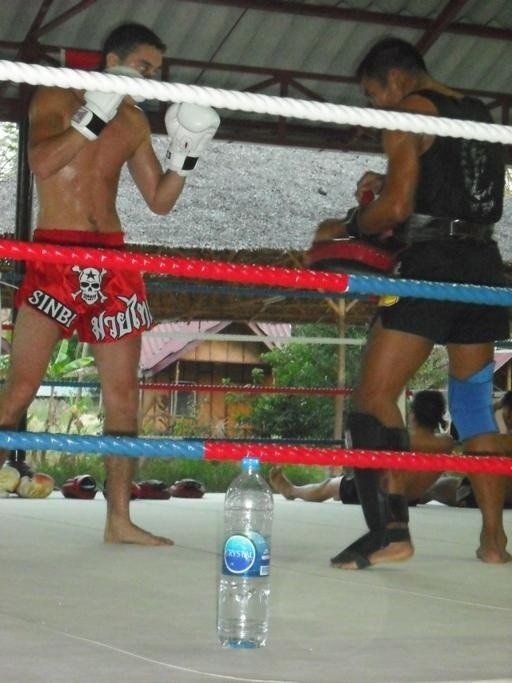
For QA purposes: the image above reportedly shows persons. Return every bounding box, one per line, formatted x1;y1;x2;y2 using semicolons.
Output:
311;35;512;574
268;389;458;507
0;21;221;548
416;389;511;509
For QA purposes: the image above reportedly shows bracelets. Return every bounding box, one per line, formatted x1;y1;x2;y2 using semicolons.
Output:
344;205;381;240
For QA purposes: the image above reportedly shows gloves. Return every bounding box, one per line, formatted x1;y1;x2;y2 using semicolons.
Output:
163;100;222;175
69;66;149;139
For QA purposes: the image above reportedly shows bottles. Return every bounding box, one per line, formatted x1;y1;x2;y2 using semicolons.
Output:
212;457;274;649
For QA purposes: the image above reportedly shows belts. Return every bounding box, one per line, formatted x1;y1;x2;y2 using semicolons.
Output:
404;211;495;241
